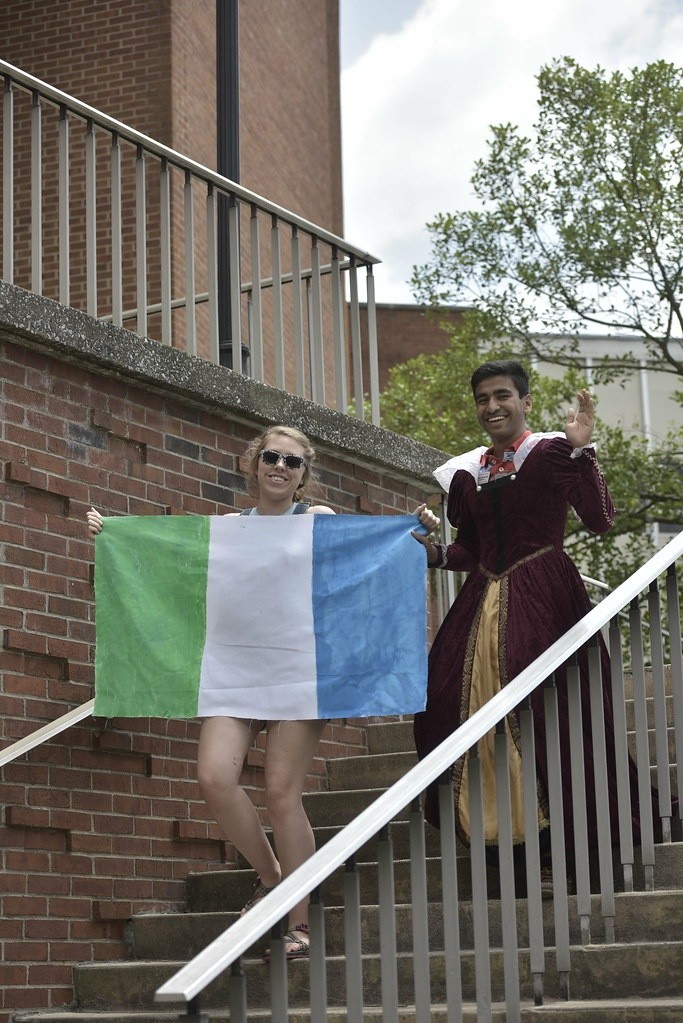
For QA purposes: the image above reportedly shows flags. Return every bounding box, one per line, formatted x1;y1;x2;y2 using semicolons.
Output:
96;514;428;720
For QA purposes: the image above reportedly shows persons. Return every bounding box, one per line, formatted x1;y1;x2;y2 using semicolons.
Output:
411;360;677;895
87;426;442;959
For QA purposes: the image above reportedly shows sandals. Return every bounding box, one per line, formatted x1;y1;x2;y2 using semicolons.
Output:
237;875;275;918
262;922;311;960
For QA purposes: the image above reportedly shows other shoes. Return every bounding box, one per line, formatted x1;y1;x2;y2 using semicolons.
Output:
541;866;575;897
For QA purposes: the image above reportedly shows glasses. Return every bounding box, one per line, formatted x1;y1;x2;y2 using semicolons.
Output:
258;448;307;470
